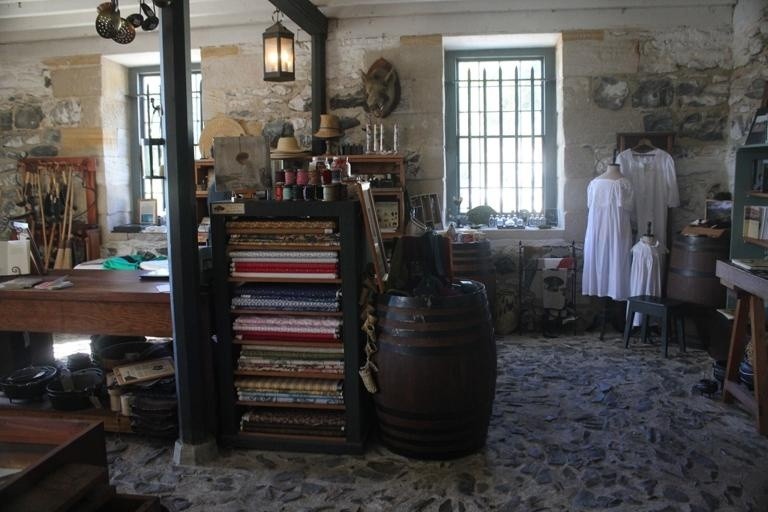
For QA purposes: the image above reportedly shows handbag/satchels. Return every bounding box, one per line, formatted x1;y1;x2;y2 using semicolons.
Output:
103;251;167;270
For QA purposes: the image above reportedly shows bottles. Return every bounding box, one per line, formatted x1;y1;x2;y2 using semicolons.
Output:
489;213;546;228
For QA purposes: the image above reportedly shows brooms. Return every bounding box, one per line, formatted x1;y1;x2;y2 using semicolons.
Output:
53;167;74;270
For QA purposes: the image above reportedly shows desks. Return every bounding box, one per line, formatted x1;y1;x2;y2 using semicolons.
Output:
0;268;178;442
0;408;168;512
713;254;768;437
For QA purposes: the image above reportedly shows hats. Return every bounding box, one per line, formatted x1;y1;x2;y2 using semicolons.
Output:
314;114;345;137
270;136;302;153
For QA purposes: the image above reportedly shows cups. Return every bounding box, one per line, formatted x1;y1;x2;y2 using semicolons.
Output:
120;394;136;417
107;387;124;411
307;155;352;185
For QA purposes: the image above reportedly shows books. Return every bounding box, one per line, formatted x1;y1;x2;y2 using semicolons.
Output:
730;106;767;274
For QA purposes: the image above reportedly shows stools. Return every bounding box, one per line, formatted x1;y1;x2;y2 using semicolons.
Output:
622;295;688;359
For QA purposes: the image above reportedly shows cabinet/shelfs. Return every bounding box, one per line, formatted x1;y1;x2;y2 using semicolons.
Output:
729;105;767;271
206;196;367;460
196;160;215;216
315;151;419;246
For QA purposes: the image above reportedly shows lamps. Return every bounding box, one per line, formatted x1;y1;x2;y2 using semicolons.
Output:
258;6;298;82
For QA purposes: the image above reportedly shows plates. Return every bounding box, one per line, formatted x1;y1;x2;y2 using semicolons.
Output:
139;260;169;271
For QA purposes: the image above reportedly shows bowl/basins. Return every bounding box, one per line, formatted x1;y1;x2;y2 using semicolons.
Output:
404;217;427;236
0;364;58;400
46;366;105;410
98;341;155;370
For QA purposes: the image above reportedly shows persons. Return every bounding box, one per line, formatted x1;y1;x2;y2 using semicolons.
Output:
580;148;637;343
627;221;670;345
236;151;261;190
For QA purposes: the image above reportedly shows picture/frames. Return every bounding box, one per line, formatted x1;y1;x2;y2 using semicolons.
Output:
136;198;158;227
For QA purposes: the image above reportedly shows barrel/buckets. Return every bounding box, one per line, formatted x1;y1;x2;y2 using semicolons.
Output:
664;235;730;343
371;278;497;461
450;239;497;325
